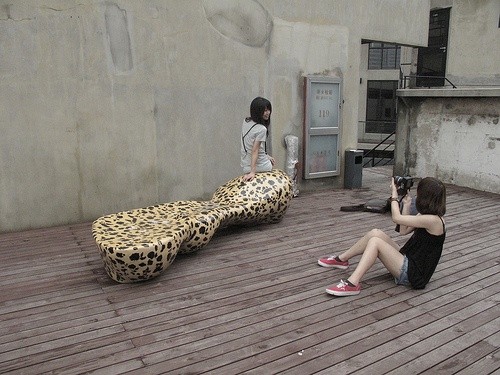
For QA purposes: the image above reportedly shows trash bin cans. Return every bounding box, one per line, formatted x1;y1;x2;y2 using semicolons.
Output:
344;150;364;190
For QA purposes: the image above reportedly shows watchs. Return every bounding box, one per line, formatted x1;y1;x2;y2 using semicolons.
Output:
391;197;400;203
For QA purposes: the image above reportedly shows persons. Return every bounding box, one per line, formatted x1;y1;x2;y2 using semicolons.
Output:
240;97;276;181
317;177;447;296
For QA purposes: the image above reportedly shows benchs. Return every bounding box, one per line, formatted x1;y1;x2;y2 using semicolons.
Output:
91;168;300;283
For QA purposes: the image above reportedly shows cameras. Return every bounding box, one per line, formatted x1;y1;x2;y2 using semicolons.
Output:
393;175;414;196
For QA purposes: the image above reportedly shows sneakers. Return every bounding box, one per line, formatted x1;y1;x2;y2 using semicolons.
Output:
326;279;360;295
318;256;349;269
